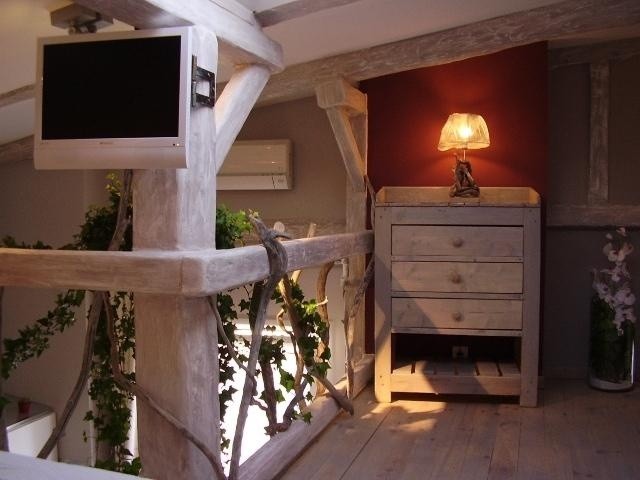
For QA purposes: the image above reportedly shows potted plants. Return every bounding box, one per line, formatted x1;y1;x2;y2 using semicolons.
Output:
18;395;32;415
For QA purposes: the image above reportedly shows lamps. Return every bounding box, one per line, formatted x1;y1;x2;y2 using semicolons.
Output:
438;113;491;198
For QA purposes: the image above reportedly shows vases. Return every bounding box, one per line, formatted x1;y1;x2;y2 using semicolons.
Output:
589;301;635;393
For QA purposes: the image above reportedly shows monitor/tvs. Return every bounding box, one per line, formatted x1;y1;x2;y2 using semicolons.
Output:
32;26;194;172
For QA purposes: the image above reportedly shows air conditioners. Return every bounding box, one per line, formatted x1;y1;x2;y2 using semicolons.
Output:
215;139;293;190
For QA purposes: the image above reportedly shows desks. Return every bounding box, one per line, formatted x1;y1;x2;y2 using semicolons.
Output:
0;393;59;464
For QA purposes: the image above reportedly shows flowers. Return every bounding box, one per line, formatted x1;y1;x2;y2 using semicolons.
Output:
589;227;637;336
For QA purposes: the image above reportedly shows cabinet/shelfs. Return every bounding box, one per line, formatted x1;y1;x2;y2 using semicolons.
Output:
372;187;541;409
